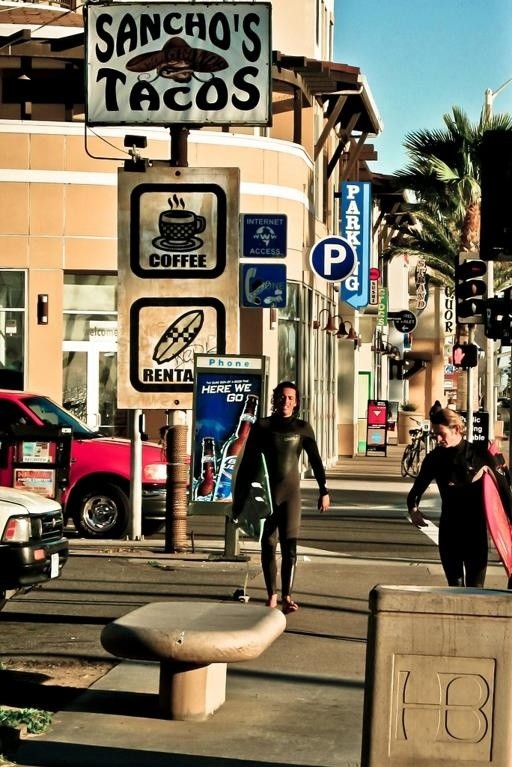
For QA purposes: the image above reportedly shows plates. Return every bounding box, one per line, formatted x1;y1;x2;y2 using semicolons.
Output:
152;238;204;254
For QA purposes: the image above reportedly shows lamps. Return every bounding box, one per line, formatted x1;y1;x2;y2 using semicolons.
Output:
312;307;358;341
371;337;401;361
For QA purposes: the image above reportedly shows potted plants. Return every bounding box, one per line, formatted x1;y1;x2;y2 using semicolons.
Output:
398;402;424;445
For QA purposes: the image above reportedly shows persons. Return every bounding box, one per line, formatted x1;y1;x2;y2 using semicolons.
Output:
447;396;457;410
232;381;330;614
429;400;442;432
407;408;512;587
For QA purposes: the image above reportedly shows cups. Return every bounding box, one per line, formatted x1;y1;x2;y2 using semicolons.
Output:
157;211;207;245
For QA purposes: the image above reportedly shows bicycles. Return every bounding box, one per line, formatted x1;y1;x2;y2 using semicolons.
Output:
400;415;436;480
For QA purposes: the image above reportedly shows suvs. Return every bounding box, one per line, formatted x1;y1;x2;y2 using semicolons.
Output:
1;486;72;609
0;388;192;539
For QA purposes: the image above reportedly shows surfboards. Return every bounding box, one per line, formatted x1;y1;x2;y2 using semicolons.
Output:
225;454;273;542
484;472;512;580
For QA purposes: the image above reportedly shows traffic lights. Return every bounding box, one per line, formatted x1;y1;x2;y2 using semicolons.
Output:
452;343;478;367
455;250;489;325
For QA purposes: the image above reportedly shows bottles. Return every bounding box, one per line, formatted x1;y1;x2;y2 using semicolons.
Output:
195;394;261;501
